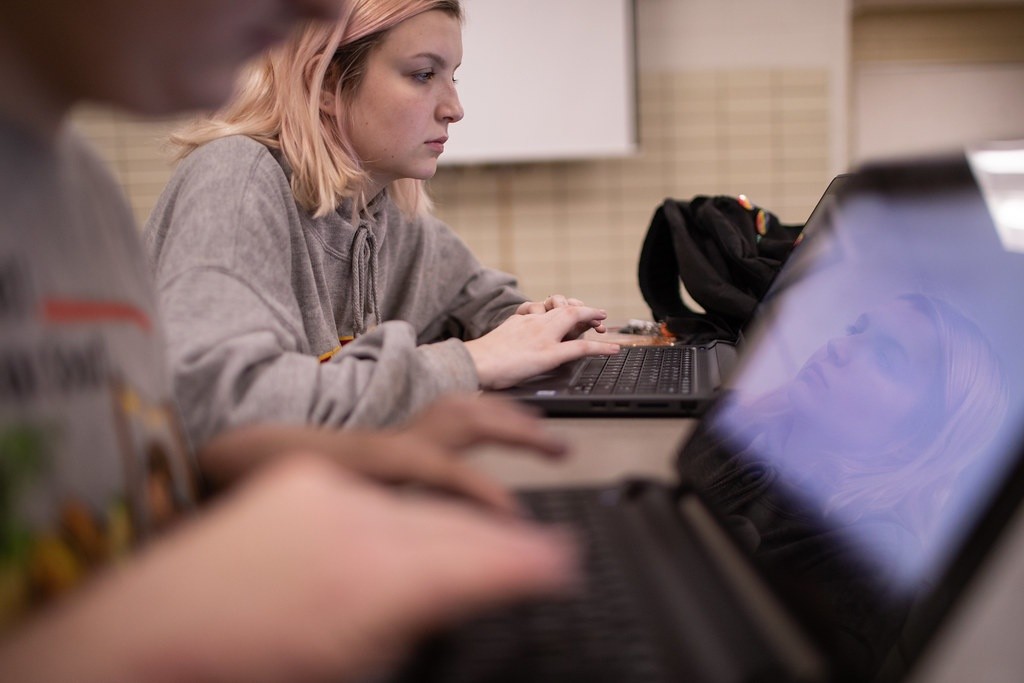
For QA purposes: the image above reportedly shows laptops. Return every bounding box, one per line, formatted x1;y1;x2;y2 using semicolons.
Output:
394;153;1024;683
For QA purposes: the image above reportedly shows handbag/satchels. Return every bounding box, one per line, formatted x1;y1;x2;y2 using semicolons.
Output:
637;196;804;343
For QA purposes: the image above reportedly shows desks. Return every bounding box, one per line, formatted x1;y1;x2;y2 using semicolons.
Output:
454;326;1024;683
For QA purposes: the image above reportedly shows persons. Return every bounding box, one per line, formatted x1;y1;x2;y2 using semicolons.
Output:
0;0;621;683
